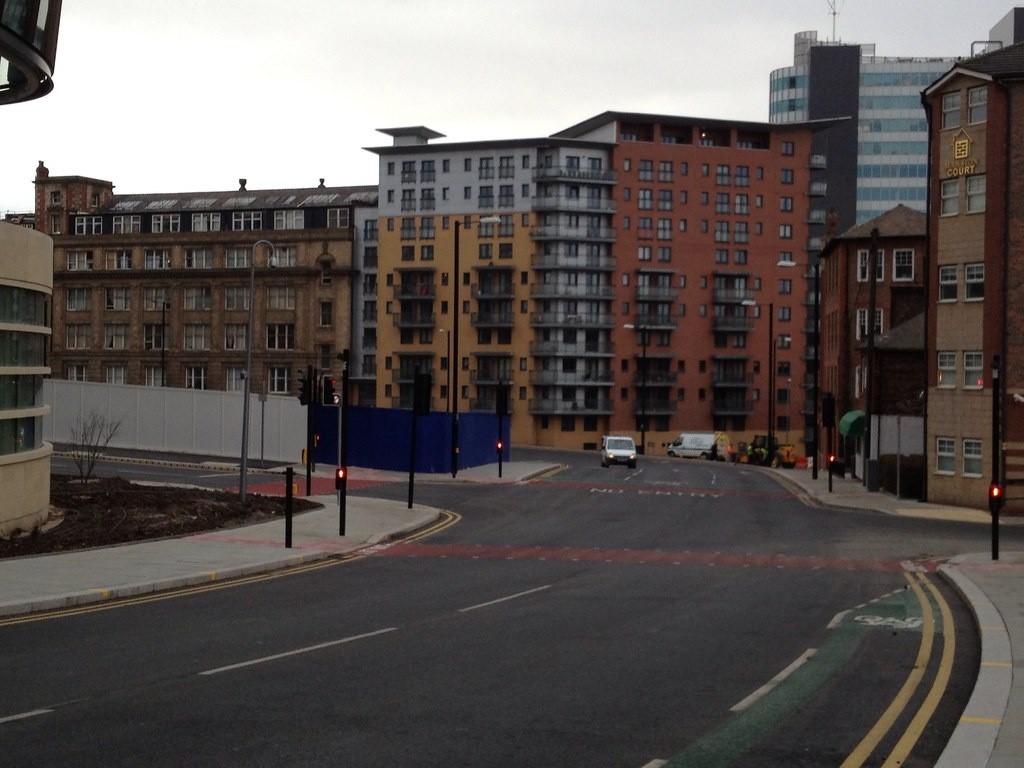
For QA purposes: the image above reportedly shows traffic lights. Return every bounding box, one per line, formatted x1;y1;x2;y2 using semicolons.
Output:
496;440;504;454
829;455;835;464
298;377;309;406
335;469;347;491
321;374;340;408
989;484;1002;508
313;435;320;448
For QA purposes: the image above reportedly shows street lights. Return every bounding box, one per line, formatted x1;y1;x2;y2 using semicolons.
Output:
439;329;450;412
625;324;646;455
775;260;821;481
239;241;278;509
741;299;775;466
450;216;501;478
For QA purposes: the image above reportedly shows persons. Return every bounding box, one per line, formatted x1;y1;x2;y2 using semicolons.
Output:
710;440;755;464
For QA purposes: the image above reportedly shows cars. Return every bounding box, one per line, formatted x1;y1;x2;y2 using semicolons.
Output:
601;435;637;469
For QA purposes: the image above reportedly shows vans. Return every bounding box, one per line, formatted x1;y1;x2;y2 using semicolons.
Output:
666;432;731;463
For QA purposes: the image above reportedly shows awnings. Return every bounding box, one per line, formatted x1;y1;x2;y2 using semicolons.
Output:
839;410;866;435
